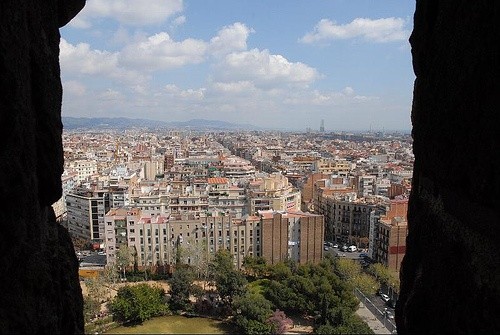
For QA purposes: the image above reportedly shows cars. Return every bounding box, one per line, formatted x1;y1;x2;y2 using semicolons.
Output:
359;253;368;257
377;292;396;308
324;241;357;252
98;251;106;256
